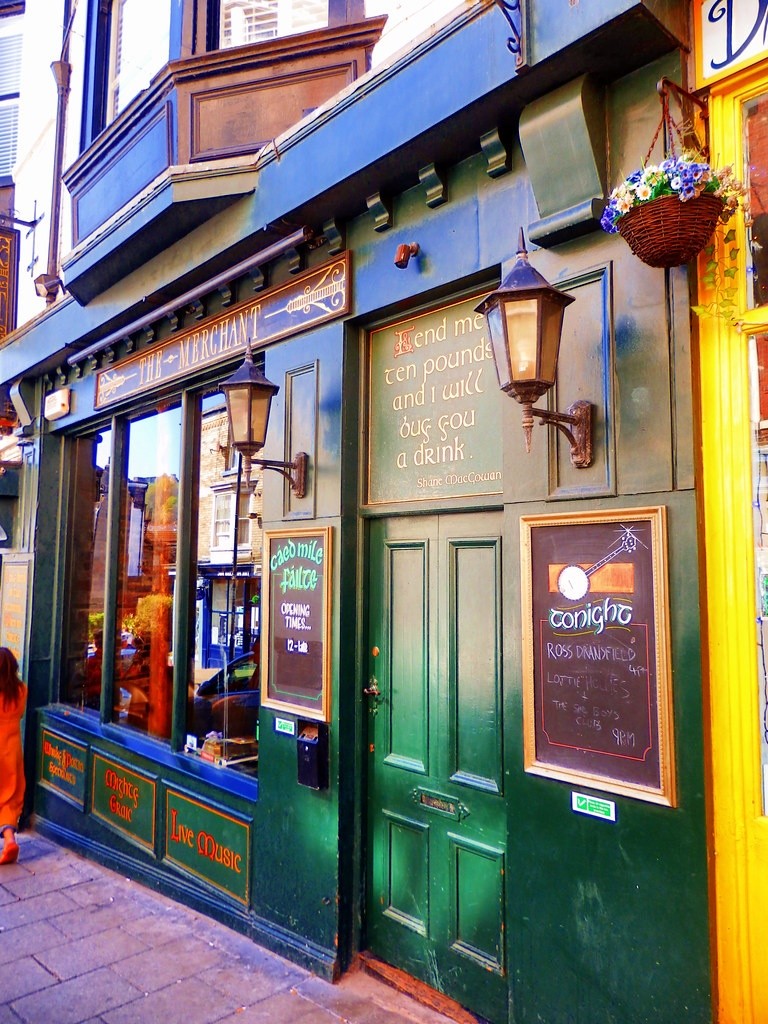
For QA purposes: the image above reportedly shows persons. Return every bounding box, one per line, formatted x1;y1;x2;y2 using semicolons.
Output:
86;631;103;710
0;647;28;863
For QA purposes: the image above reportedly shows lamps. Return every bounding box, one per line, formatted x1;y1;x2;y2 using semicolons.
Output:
218;338;308;499
393;241;420;268
34;274;66;298
473;226;594;469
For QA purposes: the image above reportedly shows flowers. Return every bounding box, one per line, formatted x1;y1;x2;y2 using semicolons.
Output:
599;155;744;239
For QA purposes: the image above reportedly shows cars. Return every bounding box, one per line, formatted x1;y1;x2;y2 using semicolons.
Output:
195;648;260;741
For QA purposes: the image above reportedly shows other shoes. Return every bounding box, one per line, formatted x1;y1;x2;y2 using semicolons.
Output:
0;842;19;865
